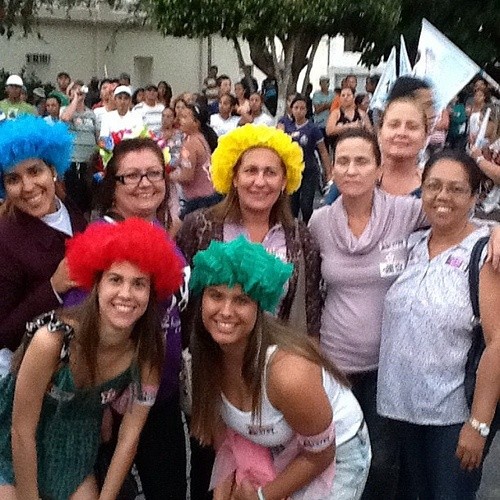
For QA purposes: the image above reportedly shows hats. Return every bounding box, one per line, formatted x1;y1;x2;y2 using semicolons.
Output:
320;75;330;83
113;85;132;98
65;79;85;98
120;72;130;78
56;70;72;81
387;76;429;100
6;74;23;87
34;87;46;98
21;86;28;94
144;83;159;91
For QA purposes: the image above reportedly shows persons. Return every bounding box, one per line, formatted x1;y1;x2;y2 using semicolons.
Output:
0;216;186;500
94;137;191;500
307;127;500;500
179;232;372;500
376;149;500;500
0;111;91;353
0;64;500;222
174;123;327;500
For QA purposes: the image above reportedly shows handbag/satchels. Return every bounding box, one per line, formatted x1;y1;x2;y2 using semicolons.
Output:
463;236;500;435
429;129;445;145
457;120;470;135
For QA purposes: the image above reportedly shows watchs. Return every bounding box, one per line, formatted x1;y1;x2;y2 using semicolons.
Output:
468;416;491;438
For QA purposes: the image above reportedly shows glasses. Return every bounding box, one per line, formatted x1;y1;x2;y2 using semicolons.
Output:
115;169;165;184
419;182;471;200
147;88;157;92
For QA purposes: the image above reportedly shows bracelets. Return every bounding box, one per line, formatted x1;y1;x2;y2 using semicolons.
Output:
477;155;484;164
257;486;264;500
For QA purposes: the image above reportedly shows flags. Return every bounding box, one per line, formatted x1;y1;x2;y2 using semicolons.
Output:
368;17;481;158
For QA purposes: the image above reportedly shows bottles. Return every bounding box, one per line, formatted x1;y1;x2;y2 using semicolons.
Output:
80;84;89;94
